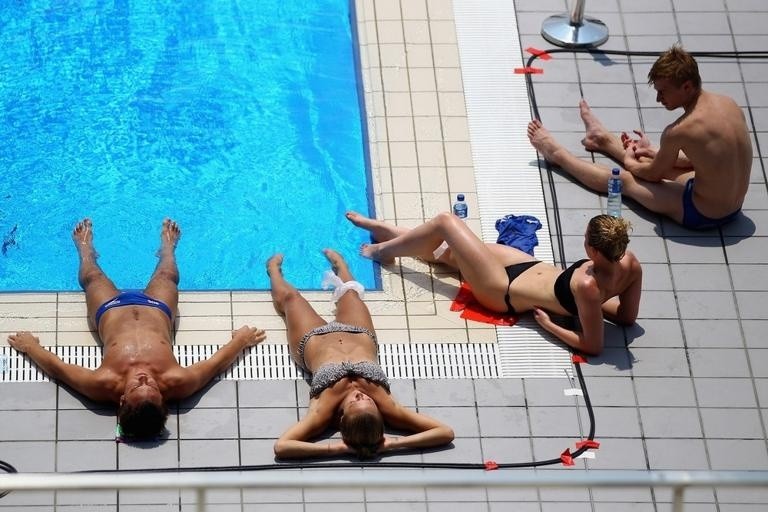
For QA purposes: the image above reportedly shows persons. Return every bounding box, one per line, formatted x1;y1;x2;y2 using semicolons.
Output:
265;247;456;460
527;41;754;232
7;214;269;443
342;211;644;357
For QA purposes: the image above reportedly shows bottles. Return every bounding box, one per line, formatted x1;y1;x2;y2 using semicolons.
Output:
452;192;470;228
604;168;627;223
